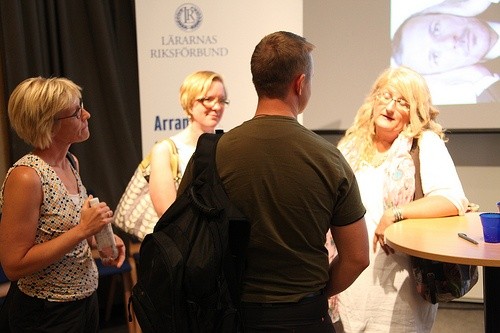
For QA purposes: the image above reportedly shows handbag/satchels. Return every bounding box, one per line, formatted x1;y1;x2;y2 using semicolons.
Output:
410;137;479;305
112;137;182;243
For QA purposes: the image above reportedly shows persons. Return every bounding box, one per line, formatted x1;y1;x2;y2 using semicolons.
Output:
175;31;370;333
392;0;500;103
0;77;126;333
150;70;229;219
324;65;469;333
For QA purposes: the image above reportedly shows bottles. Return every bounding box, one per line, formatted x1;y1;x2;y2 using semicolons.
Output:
90;198;118;261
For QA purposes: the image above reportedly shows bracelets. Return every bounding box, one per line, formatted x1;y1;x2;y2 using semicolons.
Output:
392;206;403;221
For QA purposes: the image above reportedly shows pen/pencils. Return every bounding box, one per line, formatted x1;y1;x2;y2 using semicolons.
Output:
458;233;478;244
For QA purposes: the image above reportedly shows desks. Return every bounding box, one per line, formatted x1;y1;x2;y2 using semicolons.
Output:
385;211;500;333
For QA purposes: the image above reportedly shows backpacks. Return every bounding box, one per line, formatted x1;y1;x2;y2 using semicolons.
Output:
128;129;250;333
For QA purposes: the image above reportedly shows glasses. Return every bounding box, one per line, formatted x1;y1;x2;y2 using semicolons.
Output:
193;96;230;109
54;102;83;121
375;88;410;112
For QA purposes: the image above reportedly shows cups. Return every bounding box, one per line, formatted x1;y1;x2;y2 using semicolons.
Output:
479;213;500;243
